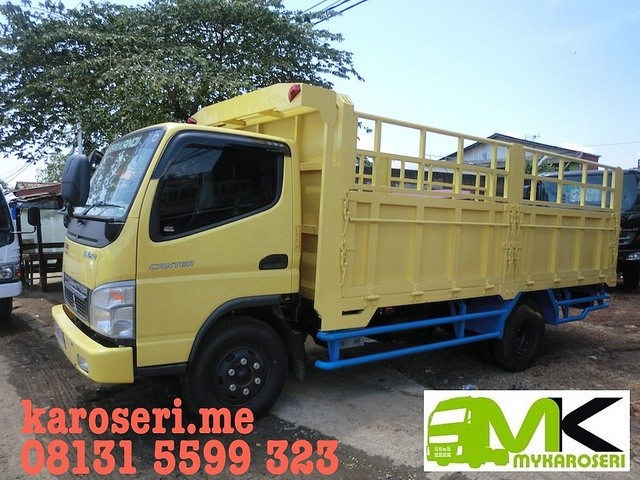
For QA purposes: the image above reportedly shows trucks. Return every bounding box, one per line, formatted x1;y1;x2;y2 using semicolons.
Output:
51;84;625;428
535;169;639;288
0;182;41;319
